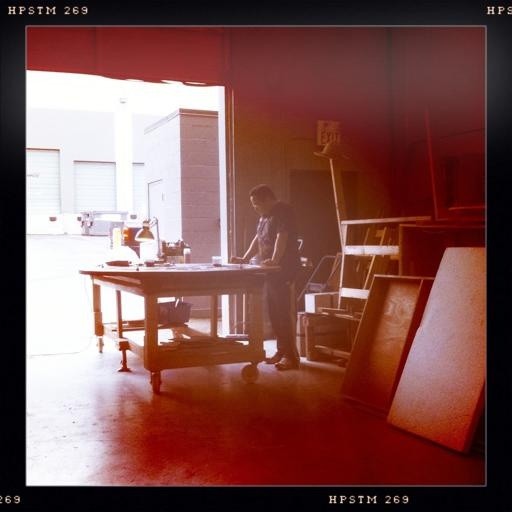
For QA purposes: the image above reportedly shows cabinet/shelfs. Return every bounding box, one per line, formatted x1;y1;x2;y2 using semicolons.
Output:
184;245;191;264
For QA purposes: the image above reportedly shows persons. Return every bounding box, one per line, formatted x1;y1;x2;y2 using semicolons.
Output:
229;184;301;371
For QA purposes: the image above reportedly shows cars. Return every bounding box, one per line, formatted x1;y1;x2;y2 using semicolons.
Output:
212;256;222;267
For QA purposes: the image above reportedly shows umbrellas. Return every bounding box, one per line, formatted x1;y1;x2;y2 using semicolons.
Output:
265;352;281;363
275;355;300;370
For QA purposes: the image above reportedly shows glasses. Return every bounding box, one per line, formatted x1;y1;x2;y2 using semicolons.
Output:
133;216;166;262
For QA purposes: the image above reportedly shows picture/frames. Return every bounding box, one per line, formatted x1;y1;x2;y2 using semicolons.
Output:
78;259;281;394
